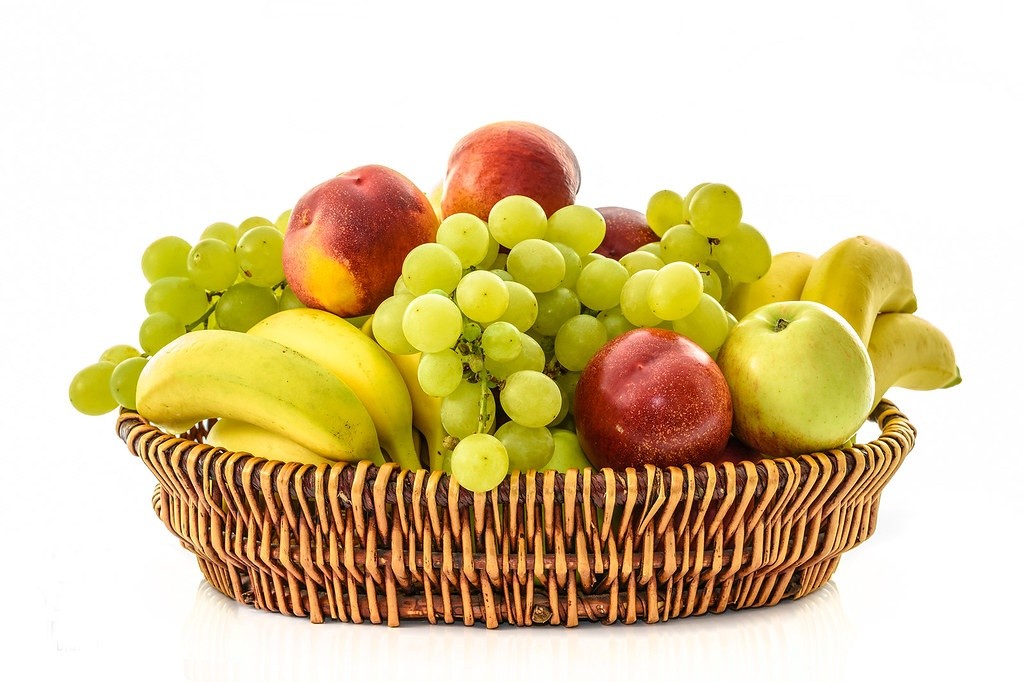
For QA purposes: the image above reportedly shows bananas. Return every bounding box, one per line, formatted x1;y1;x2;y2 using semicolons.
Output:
724;236;962;425
133;308;449;472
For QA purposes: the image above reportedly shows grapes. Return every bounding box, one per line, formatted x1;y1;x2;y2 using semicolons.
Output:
65;211;369;416
370;182;771;532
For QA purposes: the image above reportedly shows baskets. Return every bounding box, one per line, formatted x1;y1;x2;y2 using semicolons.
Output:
115;397;918;629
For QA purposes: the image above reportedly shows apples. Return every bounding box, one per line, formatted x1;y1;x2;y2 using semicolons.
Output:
713;299;877;453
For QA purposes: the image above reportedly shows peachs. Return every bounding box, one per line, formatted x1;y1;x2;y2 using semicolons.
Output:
282;119;662;317
573;326;735;472
715;436;753;467
